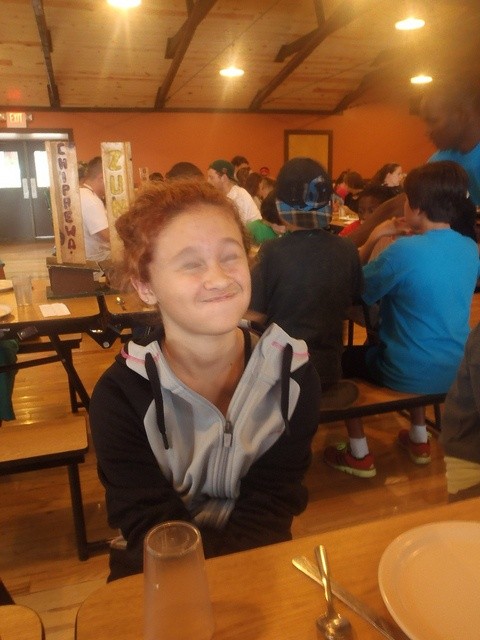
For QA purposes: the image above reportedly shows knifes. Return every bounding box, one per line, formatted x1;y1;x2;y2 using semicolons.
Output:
293;555;412;640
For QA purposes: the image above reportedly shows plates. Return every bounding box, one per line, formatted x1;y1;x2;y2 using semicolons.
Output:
377;519;480;640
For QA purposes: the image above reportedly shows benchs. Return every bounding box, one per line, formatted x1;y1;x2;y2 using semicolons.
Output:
316;374;449;432
13;333;82;413
0;416;90;560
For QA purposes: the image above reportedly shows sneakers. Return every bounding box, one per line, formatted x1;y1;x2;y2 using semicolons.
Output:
325;441;376;479
395;429;432;466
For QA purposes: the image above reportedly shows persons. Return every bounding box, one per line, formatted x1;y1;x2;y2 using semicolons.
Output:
82;180;322;584
250;157;366;409
324;159;480;479
208;155;285;241
418;73;480;210
76;154;109;260
336;162;408;210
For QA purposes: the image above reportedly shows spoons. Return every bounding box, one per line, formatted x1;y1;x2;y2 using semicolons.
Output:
116;296;128;312
315;543;353;640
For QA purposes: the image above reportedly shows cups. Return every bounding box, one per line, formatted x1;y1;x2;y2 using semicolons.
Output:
11;273;32;307
139;523;217;640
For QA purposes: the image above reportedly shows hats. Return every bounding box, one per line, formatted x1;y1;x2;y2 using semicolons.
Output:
210;158;239;181
275;158;334;229
